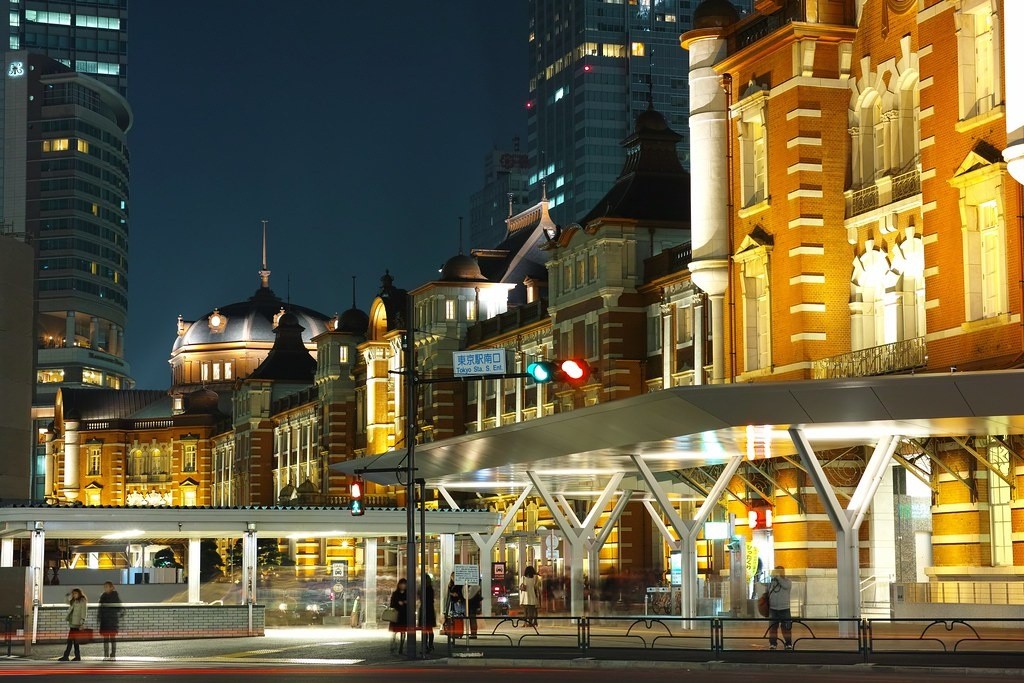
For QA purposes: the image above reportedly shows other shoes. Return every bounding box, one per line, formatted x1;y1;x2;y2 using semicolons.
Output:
58;657;69;661
469;634;477;639
103;656;116;661
786;644;792;649
769;644;776;649
70;656;80;661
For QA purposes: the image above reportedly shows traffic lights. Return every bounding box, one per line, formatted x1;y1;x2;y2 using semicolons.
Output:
349;482;364;516
527;359;589;383
748;507;773;530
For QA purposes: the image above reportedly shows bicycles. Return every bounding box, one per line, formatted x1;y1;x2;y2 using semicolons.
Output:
650;570;682;615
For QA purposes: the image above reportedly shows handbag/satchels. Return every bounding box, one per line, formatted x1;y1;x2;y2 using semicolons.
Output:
758;591;769;617
519;576;526;591
382;607;398;622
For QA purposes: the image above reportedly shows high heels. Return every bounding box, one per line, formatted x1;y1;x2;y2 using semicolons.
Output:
522;622;538;627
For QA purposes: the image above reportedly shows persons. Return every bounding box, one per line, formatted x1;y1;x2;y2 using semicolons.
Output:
468;577;484;639
389;578;407;654
97;582;125;661
443;571;466;647
767;566;793;650
519;566;539;628
58;588;87;661
416;573;437;649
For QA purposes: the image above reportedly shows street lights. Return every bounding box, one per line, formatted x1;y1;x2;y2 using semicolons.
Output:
243;521;256;635
29;519;47;643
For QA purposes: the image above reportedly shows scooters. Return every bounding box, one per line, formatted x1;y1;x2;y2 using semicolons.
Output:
496;593;510;614
